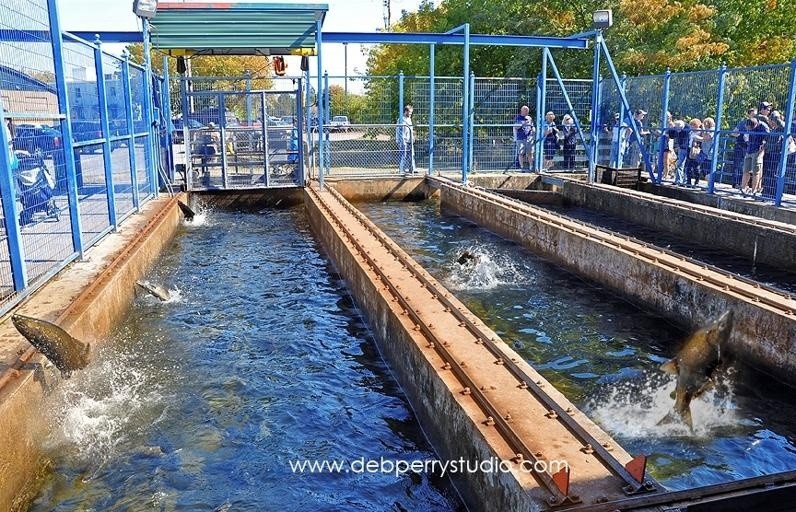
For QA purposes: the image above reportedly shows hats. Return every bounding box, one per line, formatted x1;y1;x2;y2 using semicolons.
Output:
634;109;648;115
614;112;620;119
759;101;773;109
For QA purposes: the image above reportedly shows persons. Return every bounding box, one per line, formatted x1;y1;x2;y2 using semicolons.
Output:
2;110;18;170
602;108;716;189
544;110;559;171
513;105;535;173
731;100;796;198
205;121;220;138
396;104;419;174
562;114;578;172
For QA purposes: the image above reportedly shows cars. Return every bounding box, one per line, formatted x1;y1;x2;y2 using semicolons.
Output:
271;116;293;124
15;120;142;158
173;106;262;144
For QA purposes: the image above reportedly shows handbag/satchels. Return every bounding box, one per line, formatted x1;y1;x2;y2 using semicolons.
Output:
689;146;708;165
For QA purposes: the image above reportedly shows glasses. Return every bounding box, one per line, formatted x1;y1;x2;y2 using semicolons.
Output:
566;117;571;121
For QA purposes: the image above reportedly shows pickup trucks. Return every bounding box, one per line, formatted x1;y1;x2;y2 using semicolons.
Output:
330;116;350;129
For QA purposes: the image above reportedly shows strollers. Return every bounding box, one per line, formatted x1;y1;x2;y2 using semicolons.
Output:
18;157;60;222
